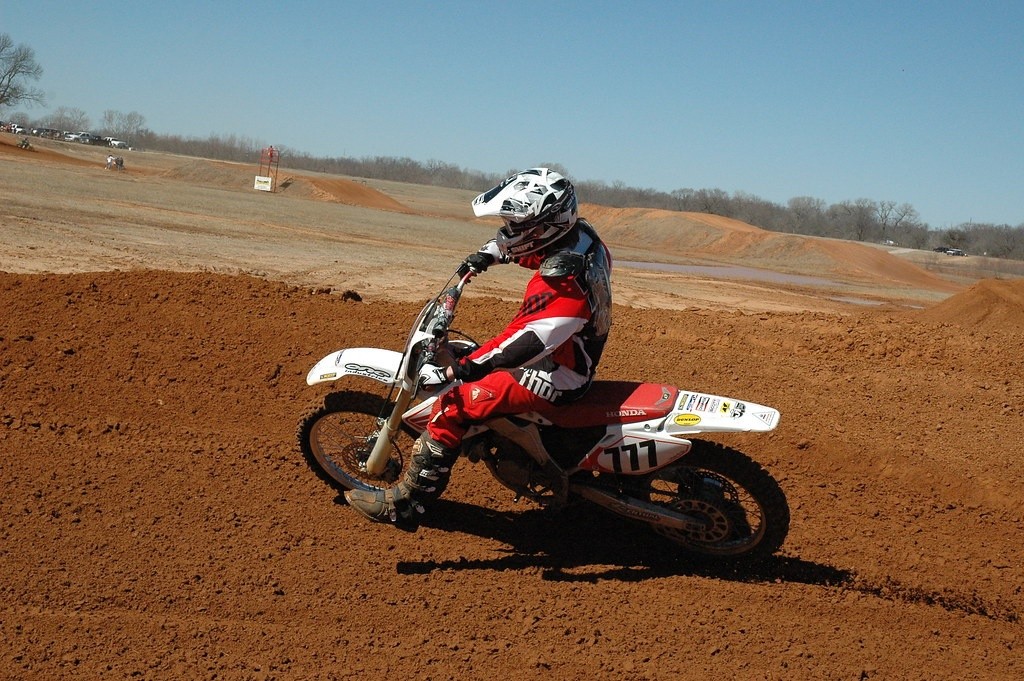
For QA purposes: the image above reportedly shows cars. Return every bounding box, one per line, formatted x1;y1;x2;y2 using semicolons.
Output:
933;247;963;256
0;122;127;149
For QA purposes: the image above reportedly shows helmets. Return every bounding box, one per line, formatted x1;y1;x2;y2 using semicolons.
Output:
472;167;577;257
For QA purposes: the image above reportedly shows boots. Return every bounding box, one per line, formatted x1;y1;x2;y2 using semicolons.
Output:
344;429;462;532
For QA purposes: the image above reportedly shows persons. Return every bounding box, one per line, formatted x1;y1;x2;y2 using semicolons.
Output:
9;124;11;131
268;145;273;158
343;168;611;534
105;155;123;172
22;138;29;148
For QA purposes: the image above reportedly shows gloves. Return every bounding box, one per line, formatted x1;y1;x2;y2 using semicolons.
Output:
478;239;511;267
419;363;452;393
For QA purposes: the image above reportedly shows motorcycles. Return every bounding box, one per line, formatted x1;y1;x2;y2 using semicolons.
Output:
296;238;790;568
